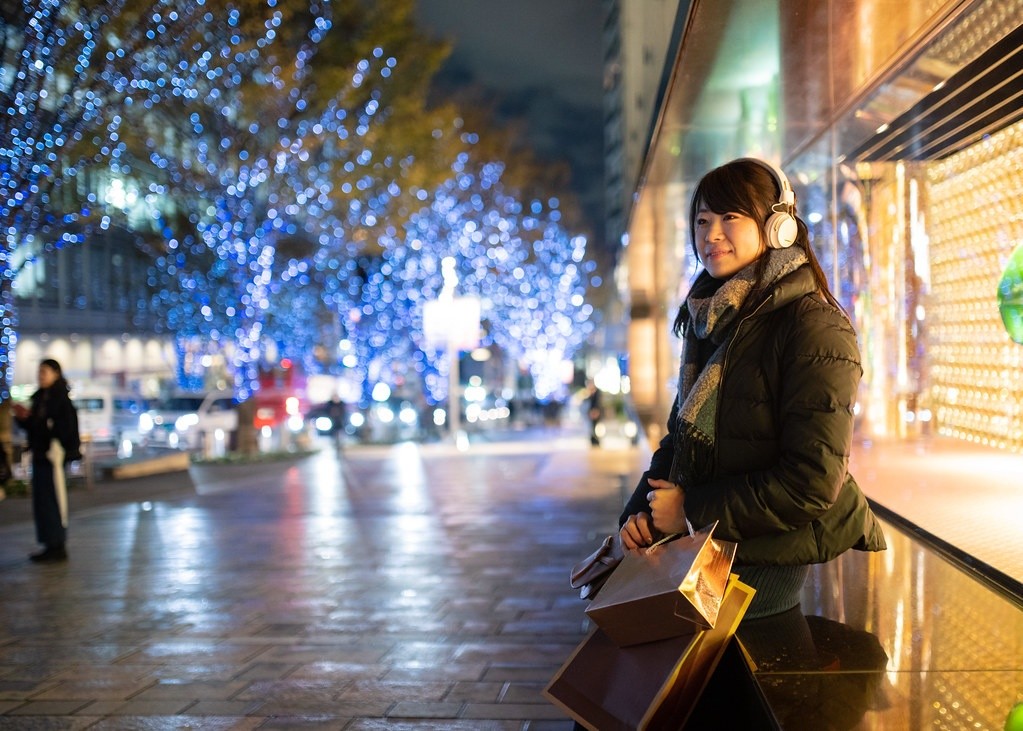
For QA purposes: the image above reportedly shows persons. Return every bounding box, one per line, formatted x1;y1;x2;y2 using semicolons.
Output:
585;379;606;447
570;156;886;731
11;359;84;564
323;391;349;451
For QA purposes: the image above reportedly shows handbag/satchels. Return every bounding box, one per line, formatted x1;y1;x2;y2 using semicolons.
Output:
584;517;738;647
541;518;756;731
571;532;628;599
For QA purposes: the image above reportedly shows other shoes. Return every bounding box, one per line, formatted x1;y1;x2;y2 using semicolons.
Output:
30;544;67;562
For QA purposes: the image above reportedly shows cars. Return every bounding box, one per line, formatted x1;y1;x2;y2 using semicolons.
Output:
0;381;426;485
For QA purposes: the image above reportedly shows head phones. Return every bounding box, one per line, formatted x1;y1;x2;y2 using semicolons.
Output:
731;156;798;249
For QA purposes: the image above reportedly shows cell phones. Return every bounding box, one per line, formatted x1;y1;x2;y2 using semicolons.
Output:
13;404;26;415
